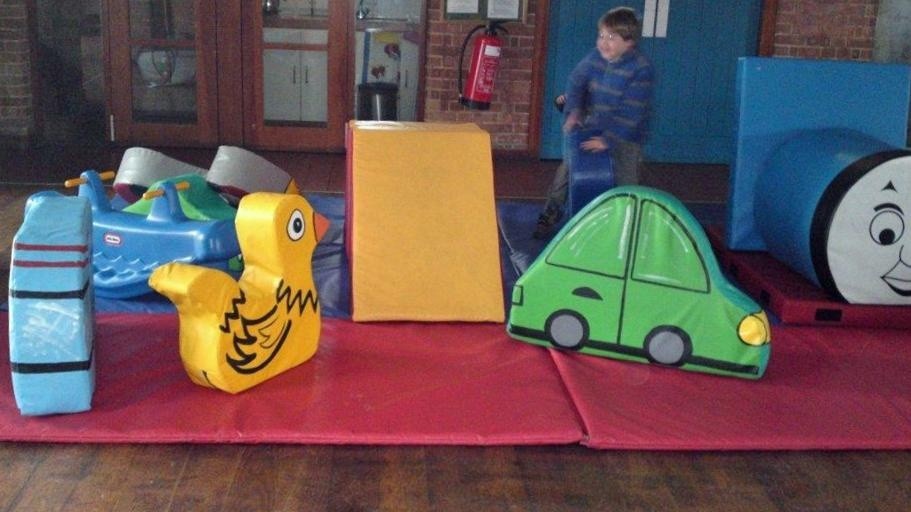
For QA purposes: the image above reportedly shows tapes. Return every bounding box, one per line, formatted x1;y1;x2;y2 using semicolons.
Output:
112;147;296;201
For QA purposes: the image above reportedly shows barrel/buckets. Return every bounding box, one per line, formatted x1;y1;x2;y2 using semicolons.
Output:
356;81;399;121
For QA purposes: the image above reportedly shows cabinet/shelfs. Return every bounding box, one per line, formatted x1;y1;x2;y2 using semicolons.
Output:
262;27;365;122
366;29;418;122
132;48;195;113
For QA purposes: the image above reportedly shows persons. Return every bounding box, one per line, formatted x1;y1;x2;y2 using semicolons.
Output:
538;7;653;228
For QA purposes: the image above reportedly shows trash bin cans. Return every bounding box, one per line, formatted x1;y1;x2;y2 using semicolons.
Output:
356;82;399;122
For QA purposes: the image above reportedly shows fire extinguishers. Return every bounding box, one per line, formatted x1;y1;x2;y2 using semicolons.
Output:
456;19;511;111
